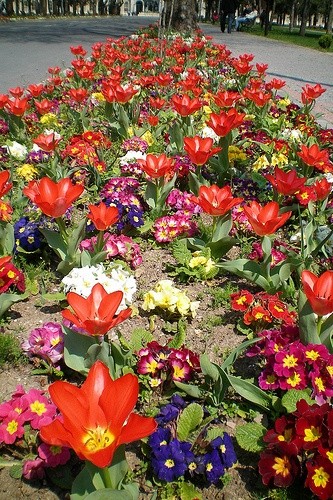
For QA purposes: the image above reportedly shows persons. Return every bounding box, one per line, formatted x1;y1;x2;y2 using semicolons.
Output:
127;9;139;17
211;2;270;35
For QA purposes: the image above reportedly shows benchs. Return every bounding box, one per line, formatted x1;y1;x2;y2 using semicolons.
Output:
239;16;258;33
